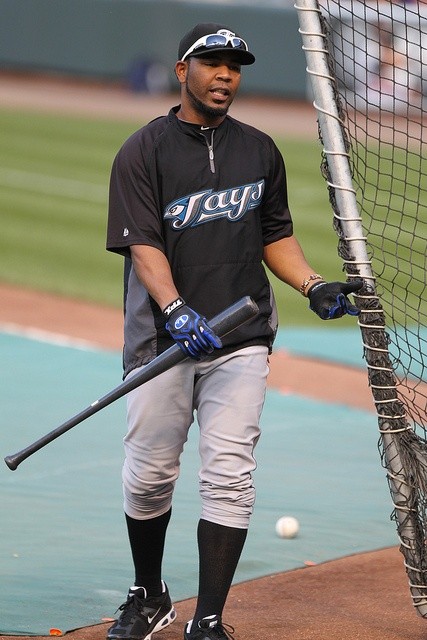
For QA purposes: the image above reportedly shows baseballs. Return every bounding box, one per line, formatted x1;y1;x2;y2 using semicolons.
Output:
276;514;300;540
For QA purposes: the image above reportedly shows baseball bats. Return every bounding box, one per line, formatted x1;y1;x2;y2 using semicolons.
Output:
5;295;261;473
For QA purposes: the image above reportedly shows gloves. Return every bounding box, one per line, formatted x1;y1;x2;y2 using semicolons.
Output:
308;281;364;320
162;298;222;362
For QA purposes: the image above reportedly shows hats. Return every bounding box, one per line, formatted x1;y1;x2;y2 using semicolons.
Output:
178;24;255;63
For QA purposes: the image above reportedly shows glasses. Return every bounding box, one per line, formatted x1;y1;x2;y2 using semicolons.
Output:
180;33;249;61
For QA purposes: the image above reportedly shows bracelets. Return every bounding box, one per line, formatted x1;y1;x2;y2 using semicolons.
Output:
299;274;321;297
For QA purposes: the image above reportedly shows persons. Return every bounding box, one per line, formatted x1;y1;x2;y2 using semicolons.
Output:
105;22;364;640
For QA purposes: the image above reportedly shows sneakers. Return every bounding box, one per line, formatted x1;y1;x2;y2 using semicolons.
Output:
184;615;235;640
107;579;177;640
